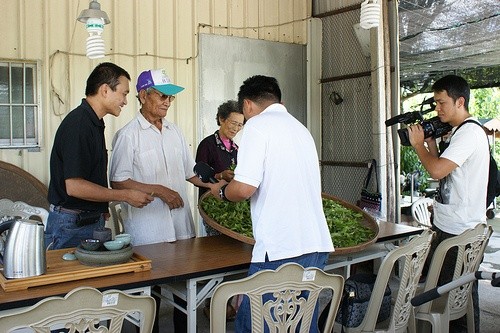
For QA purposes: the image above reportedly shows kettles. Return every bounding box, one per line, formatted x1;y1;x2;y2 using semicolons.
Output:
0;219;47;278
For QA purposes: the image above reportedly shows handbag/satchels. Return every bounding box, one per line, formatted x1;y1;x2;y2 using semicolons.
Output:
486;154;500;207
334;273;392;327
359;159;382;212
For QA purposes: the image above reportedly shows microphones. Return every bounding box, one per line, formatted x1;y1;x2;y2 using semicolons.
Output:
193;161;219;184
385;114;405;127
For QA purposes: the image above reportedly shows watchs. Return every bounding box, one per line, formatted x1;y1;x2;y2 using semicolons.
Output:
219;184;231;202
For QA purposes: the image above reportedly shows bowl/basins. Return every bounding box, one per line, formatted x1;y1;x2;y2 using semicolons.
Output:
79;227;132;251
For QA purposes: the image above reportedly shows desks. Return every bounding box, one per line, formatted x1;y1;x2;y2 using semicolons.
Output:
132;222;424;333
0;249;174;333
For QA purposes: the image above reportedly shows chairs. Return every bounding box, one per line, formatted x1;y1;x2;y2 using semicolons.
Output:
0;287;155;333
0;199;48;255
410;199;435;230
330;229;434;333
109;198;127;237
210;262;346;333
390;222;496;333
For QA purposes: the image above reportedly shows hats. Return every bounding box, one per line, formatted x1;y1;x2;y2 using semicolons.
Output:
137;70;185;96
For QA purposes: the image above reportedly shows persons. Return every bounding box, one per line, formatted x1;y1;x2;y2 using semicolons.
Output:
109;69;212;333
43;62;154;250
211;74;336;333
194;100;246;318
407;75;491;333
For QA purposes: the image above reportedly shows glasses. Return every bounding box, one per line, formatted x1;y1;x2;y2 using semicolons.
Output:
150;89;175;102
225;118;243;130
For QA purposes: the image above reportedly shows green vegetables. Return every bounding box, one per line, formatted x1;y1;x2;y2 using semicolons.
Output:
322;197;373;248
230;164;236;170
202;193;252;237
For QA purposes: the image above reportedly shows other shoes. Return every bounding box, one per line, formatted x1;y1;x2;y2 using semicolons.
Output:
227;304;237;319
203;306;210;319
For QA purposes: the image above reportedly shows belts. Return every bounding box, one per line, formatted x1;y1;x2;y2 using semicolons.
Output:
53;206;101;216
